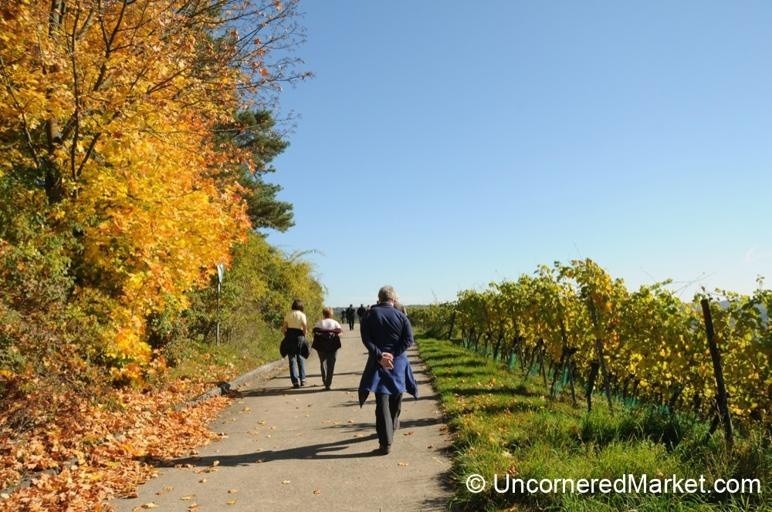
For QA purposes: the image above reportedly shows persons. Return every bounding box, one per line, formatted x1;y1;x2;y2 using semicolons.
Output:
342;301;407;330
360;285;418;455
312;308;342;389
280;299;310;388
